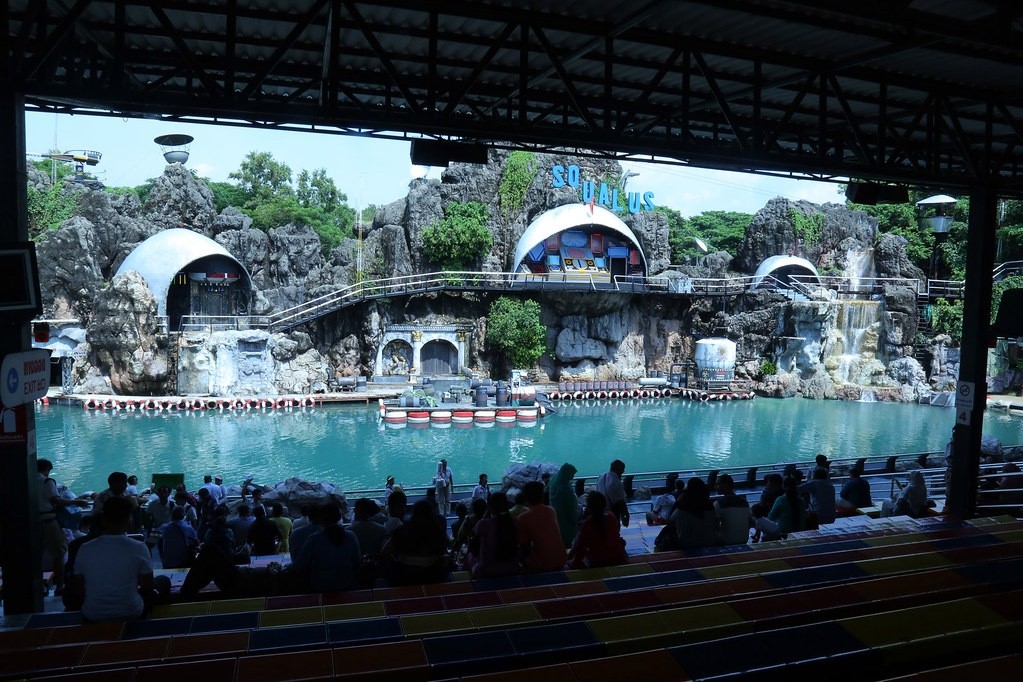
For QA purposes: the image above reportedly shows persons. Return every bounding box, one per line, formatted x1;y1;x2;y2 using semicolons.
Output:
29;454;1023;624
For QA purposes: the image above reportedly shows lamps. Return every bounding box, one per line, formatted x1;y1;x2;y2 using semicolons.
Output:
846;178;910;205
410;137;488;168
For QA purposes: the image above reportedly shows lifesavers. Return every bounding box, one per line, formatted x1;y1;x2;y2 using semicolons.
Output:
83;395;315;410
41;396;49;405
678;388;756;402
543;389;673;400
539;403;545;418
378;398;386;420
